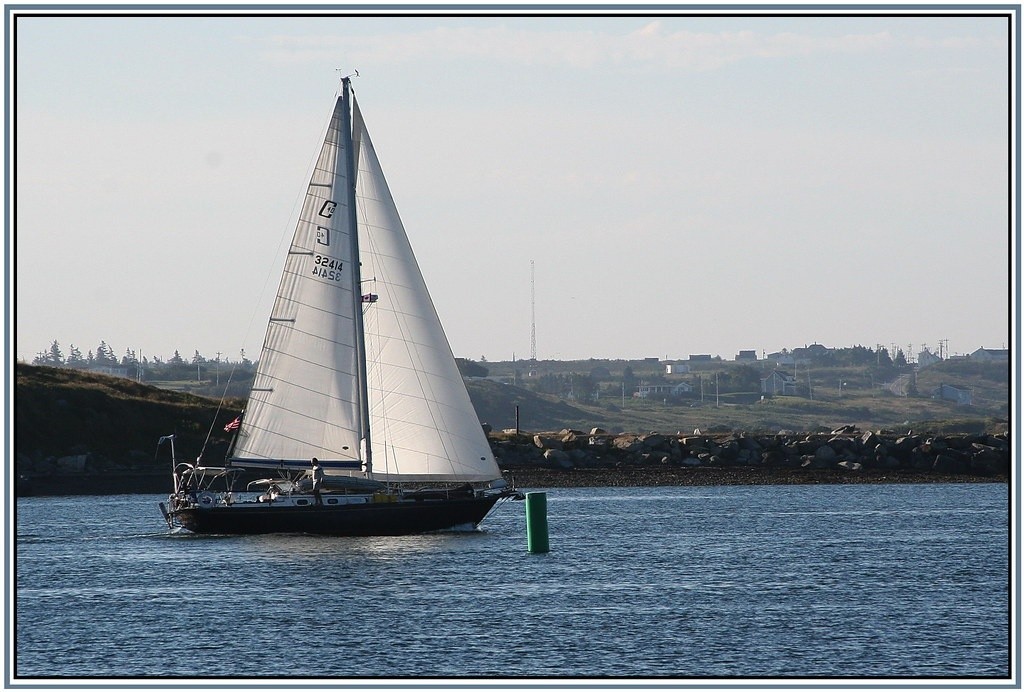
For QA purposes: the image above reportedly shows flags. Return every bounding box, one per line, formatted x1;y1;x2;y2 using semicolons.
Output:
224;415;243;433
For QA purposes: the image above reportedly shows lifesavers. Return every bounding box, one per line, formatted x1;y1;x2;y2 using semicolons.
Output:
198;491;216;509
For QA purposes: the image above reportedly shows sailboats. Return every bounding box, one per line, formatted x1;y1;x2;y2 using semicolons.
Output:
156;65;527;535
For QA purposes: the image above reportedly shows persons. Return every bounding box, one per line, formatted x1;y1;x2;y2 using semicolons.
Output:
310;458;324;506
